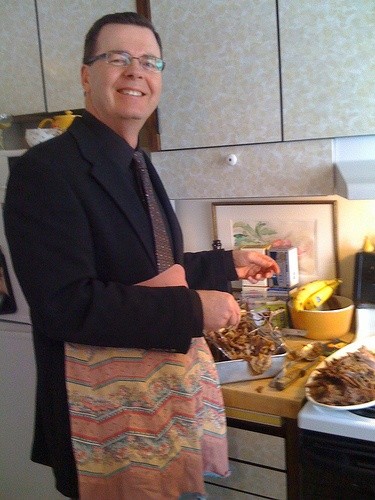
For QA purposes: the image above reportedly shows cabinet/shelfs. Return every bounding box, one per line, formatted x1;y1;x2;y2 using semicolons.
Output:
1;0;375;201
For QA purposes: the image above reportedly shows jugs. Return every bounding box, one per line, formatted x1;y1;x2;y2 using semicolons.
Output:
37;112;82;134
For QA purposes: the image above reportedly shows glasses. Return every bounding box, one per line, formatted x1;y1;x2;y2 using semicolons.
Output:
85;50;166;73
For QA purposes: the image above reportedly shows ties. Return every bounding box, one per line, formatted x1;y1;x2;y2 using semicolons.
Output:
131;148;175;276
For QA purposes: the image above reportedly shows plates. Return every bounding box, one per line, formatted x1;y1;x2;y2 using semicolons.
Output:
305;336;375;412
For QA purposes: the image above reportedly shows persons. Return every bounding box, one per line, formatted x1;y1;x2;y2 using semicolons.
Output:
3;9;281;498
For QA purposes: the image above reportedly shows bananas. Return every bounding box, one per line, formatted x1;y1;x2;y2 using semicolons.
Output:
292;279;337;312
304;279;343;310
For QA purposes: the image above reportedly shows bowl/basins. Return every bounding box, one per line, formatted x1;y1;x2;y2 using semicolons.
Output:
26;128;59;147
288;295;355;341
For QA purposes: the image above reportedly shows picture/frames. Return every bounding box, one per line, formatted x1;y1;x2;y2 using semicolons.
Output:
211;200;341;295
